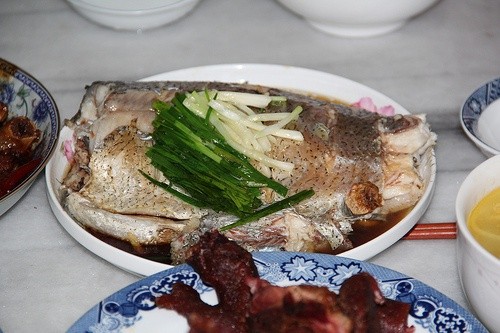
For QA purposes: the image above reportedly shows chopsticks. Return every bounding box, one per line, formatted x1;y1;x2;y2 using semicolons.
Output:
401;223;457;239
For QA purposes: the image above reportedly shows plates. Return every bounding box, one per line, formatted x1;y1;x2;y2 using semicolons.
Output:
459;78;500;158
46;63;436;278
65;252;492;333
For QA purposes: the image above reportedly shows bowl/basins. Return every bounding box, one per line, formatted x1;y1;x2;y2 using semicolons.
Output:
278;0;439;37
455;154;500;333
66;0;200;29
0;59;60;217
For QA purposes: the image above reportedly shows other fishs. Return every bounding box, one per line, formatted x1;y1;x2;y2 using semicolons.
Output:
56;80;438;256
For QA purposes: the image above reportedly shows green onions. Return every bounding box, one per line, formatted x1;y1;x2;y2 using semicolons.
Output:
138;88;315;232
